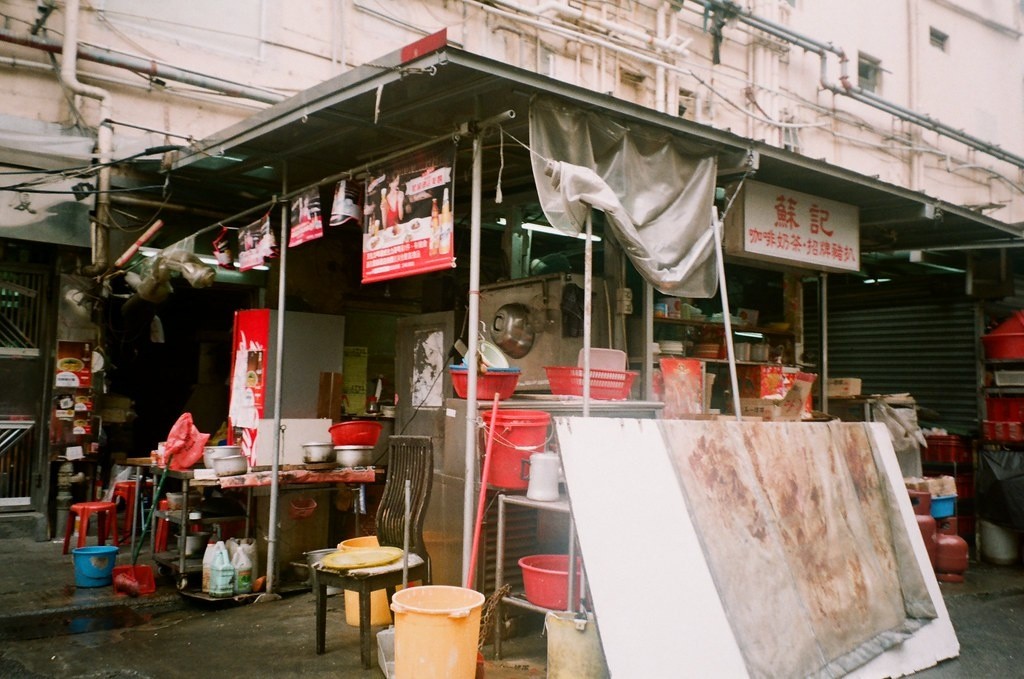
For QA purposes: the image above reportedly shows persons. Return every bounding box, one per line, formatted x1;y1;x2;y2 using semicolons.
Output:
379;170;405;230
298;194;312;224
244;228;254;252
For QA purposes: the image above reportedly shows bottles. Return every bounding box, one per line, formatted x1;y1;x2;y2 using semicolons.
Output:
203;530;259;599
366;395;378;416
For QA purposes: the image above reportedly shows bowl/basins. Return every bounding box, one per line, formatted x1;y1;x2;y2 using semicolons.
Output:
300;443;335;462
449;340;522;399
202;444;241;469
328;420;383;445
333;445;375;467
212;453;247;476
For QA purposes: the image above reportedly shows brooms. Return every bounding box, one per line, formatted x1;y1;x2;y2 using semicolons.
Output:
113;453;175;600
447;388;503;678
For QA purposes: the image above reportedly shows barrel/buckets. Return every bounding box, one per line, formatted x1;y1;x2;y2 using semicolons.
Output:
389;583;486;679
337;535;408;626
304;548;344;597
72;545;120;589
484;406;553;491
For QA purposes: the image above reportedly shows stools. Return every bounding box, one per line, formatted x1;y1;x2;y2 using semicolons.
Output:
104;482;155;545
155;499;202;552
62;501;119;554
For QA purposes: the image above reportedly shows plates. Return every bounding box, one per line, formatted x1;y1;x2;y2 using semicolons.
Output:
653;336;770;366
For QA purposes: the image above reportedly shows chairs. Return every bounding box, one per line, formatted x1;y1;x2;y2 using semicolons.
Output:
315;435;433;670
